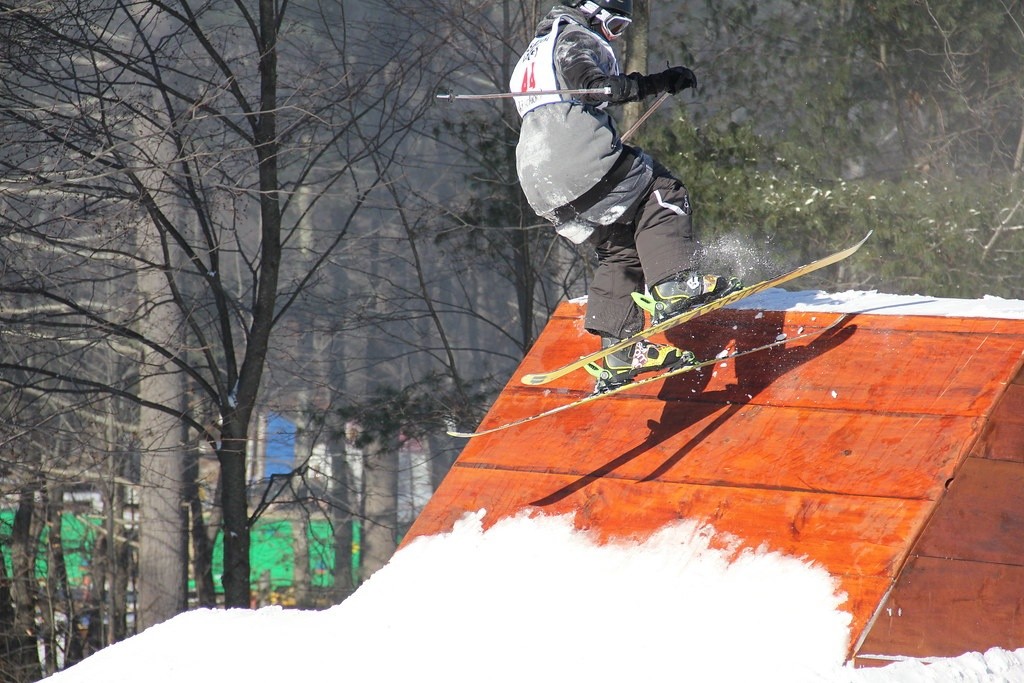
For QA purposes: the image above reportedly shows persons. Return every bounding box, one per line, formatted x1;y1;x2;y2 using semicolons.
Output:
508;0;745;392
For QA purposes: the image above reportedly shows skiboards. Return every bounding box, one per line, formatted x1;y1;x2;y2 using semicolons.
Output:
443;228;875;438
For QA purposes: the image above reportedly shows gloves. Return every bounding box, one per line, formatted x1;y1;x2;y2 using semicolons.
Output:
637;66;697;100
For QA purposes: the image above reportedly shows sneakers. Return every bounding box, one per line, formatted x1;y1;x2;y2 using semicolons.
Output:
652;272;727;325
601;331;684;380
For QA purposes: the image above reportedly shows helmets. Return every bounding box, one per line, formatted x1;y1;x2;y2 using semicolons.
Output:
568;0;632;15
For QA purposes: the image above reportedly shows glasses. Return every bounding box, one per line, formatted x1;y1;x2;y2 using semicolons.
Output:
602;13;632;38
